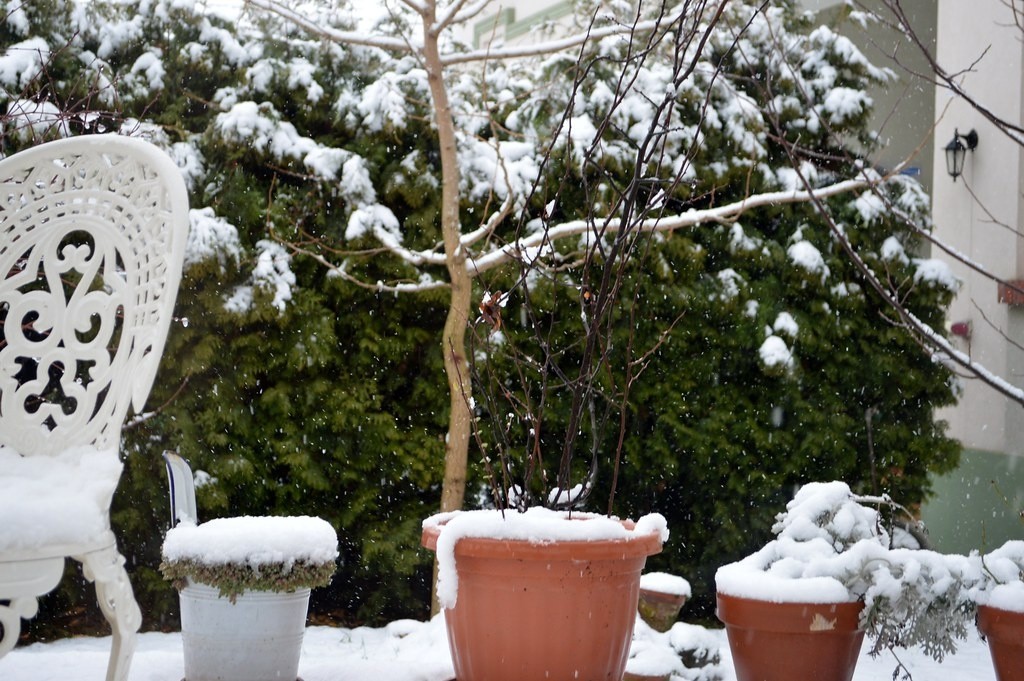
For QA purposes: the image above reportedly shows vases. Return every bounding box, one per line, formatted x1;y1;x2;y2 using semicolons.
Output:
638;588;686;633
420;508;663;681
976;605;1024;681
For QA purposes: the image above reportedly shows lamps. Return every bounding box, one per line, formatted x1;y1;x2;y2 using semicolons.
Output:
941;127;978;182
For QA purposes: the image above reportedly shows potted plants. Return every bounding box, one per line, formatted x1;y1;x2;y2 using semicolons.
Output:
715;493;976;681
158;515;337;681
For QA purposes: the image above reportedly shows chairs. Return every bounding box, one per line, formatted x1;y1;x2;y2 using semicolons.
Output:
0;134;191;681
